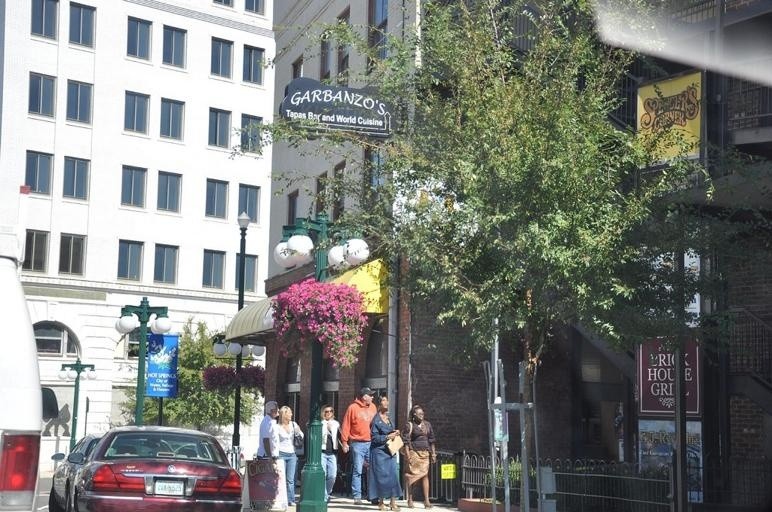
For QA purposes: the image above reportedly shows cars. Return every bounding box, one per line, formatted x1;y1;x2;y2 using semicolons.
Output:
47;432;174;512
71;425;242;512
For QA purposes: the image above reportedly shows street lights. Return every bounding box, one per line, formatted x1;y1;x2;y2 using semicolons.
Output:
232;210;250;449
114;295;172;434
274;210;369;512
58;358;97;456
209;333;266;462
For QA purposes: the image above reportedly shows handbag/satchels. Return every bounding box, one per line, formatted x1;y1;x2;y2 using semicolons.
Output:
385;431;404;457
294;433;303;447
400;422;412;455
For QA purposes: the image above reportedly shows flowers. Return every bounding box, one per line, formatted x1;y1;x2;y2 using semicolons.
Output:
271;275;368;370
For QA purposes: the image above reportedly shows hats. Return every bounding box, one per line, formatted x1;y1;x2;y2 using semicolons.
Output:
359;387;376;395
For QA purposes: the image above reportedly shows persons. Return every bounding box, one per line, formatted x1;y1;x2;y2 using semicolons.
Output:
278;406;304;508
366;394;404;510
398;404;436;509
320;405;349;503
255;400;281;458
340;387;379;506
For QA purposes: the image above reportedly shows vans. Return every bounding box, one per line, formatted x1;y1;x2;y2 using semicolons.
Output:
0;256;42;512
